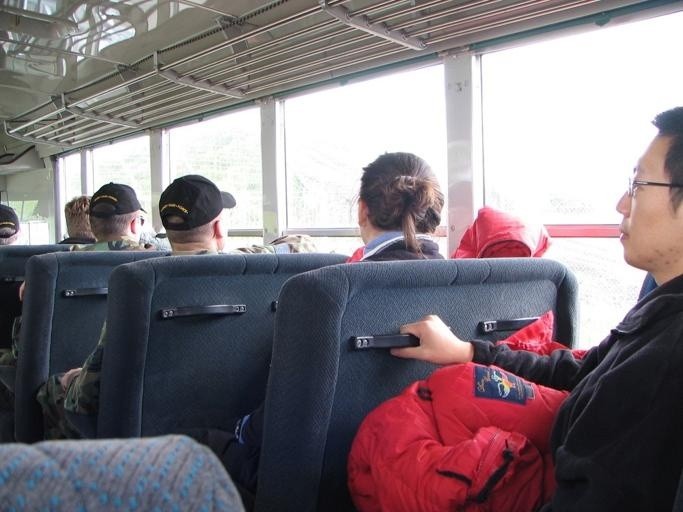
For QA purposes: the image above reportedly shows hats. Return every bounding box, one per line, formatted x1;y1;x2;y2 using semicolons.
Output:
159;175;236;230
0;204;19;238
89;182;147;217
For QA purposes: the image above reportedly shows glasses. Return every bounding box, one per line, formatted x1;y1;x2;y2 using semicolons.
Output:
628;177;683;197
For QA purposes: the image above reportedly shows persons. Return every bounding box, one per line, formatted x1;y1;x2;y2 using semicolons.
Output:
57;195;96;244
36;175;317;440
390;107;683;512
0;182;157;365
0;204;21;245
177;152;446;511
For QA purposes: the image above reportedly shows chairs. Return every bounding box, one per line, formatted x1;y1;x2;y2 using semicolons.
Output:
0;245;69;348
0;253;166;444
66;253;351;437
0;435;247;512
254;257;579;512
